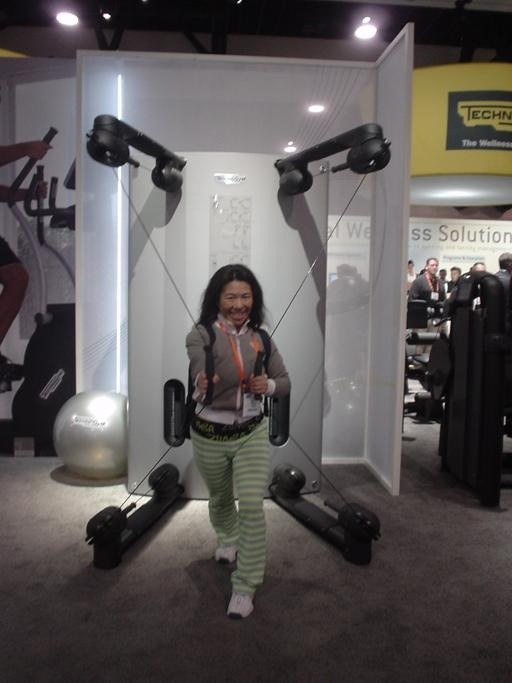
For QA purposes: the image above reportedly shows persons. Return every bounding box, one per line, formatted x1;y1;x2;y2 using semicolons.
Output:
186;264;291;620
0;140;53;381
407;252;512;354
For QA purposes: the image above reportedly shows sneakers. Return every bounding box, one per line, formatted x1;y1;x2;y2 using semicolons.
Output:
215;544;241;563
226;591;254;618
